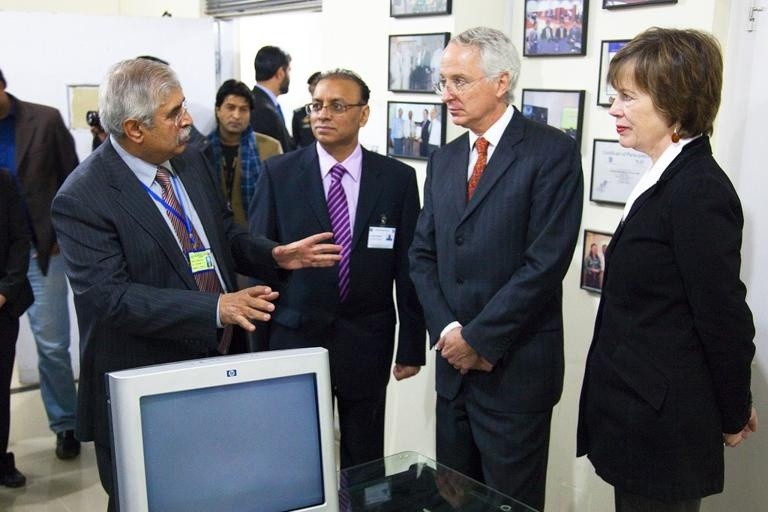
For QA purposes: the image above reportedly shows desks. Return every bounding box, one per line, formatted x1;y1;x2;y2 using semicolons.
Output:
340;451;538;512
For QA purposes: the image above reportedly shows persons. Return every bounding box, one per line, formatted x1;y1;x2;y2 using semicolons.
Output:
414;109;432;156
0;71;87;458
202;78;287;358
0;163;34;486
51;59;344;511
577;29;757;511
585;244;603;289
527;9;584;56
89;55;210;169
245;72;428;472
404;111;416;154
291;72;327;150
249;46;296;155
408;28;585;511
391;108;405;154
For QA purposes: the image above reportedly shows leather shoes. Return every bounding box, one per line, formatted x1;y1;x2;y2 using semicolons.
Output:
0;466;24;486
56;429;81;459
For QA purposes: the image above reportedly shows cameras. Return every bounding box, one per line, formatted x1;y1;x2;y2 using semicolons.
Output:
86;110;100;125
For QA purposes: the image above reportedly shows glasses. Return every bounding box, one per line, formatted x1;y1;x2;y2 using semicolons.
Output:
433;74;496;96
305;102;366;115
176;97;193;126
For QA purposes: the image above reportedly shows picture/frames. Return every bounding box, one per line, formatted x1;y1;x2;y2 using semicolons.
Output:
579;229;613;294
66;85;100;129
386;101;447;162
390;0;452;17
523;0;589;56
589;139;652;205
603;0;679;9
521;88;585;149
388;32;450;94
597;40;633;106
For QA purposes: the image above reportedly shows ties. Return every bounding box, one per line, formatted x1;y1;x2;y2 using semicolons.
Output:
464;137;489;203
325;164;351;309
338;469;350;512
158;171;233;354
278;104;287;126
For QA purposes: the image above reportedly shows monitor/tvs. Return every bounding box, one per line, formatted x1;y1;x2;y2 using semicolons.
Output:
106;348;338;512
